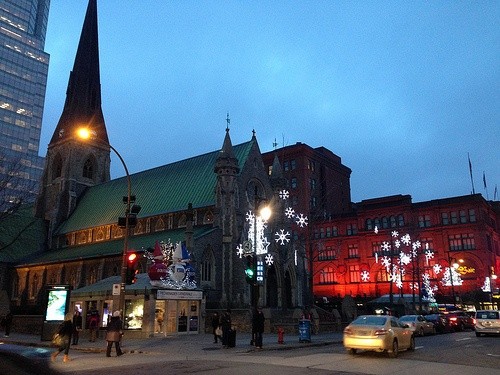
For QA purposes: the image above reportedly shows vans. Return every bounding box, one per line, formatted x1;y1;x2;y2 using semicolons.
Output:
474;310;500;336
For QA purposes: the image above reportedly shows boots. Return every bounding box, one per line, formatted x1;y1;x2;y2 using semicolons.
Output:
64;354;70;362
51;351;59;362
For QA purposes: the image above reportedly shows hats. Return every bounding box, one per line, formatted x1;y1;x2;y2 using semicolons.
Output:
113;311;120;316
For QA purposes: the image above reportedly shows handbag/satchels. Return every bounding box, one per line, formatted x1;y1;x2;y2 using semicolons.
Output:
52;334;68;345
215;326;222;335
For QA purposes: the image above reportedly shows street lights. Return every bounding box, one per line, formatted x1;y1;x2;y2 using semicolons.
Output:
75;126;132;348
250;186;273;346
447;251;459;309
487;266;497;303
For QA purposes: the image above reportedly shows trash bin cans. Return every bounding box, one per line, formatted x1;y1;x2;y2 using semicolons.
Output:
299;319;310;343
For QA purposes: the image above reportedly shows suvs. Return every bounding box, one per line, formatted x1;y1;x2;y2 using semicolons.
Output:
446;311;475;331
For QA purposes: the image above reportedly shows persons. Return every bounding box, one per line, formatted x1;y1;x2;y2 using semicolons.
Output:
51;313;74;362
302;310;310;320
72;311;82;345
253;306;264;350
3;301;16;338
213;311;223;343
86;306;101;343
105;311;125;357
311;312;316;327
220;309;231;349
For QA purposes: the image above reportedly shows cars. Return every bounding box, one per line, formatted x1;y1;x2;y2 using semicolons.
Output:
343;314;416;359
424;314;452;332
399;314;437;336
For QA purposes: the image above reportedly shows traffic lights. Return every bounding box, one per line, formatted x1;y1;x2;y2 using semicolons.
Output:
126;252;139;285
245;253;257;283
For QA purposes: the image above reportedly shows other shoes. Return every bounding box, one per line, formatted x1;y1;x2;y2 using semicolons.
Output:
213;341;218;343
106;354;111;357
72;343;77;345
118;353;123;356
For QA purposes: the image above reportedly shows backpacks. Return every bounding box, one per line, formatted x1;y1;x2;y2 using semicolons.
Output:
90;316;97;326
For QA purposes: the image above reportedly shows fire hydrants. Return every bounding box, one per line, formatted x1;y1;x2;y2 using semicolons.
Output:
278;327;284;344
